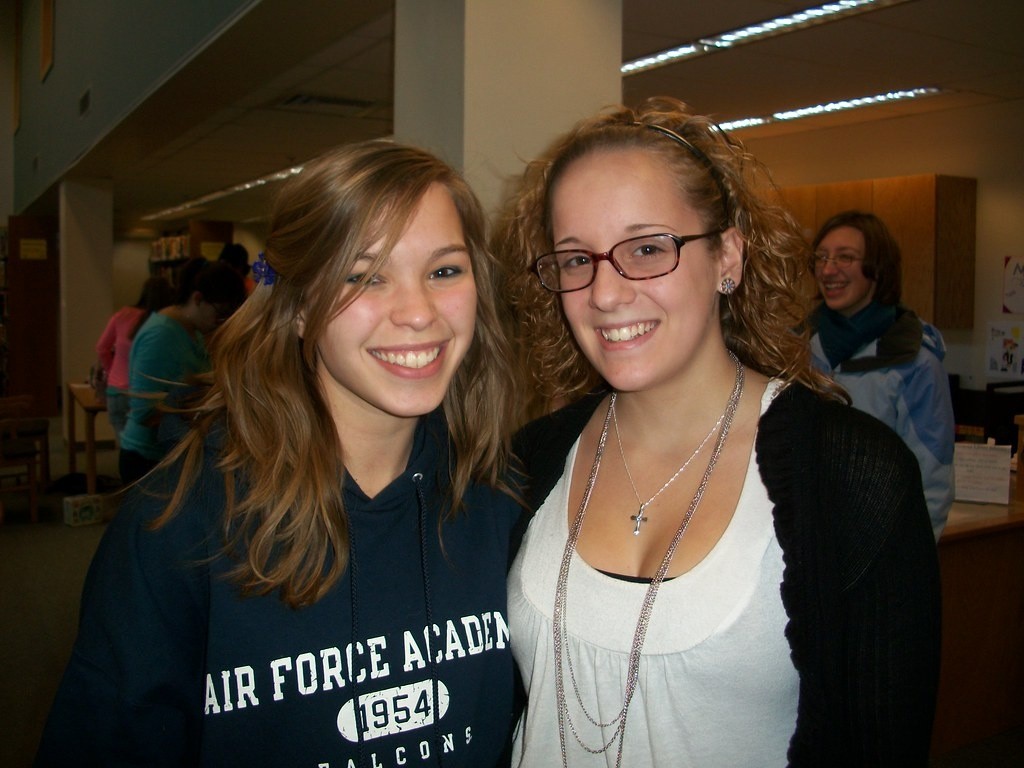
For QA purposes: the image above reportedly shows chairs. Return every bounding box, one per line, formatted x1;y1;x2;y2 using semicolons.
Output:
0;402;39;525
0;394;52;479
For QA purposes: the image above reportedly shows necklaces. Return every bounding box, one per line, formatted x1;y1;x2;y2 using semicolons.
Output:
550;350;745;768
612;405;724;536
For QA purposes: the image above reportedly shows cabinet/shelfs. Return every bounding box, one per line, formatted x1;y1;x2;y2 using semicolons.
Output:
755;184;816;309
148;218;234;291
816;170;976;331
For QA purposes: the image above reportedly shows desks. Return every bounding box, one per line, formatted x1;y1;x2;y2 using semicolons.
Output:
67;383;116;495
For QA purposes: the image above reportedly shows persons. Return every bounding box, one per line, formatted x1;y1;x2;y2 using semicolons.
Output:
787;212;954;539
92;242;257;488
481;95;937;768
33;141;546;768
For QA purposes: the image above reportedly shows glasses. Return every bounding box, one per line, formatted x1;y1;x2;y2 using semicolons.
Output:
807;254;869;271
530;231;723;294
